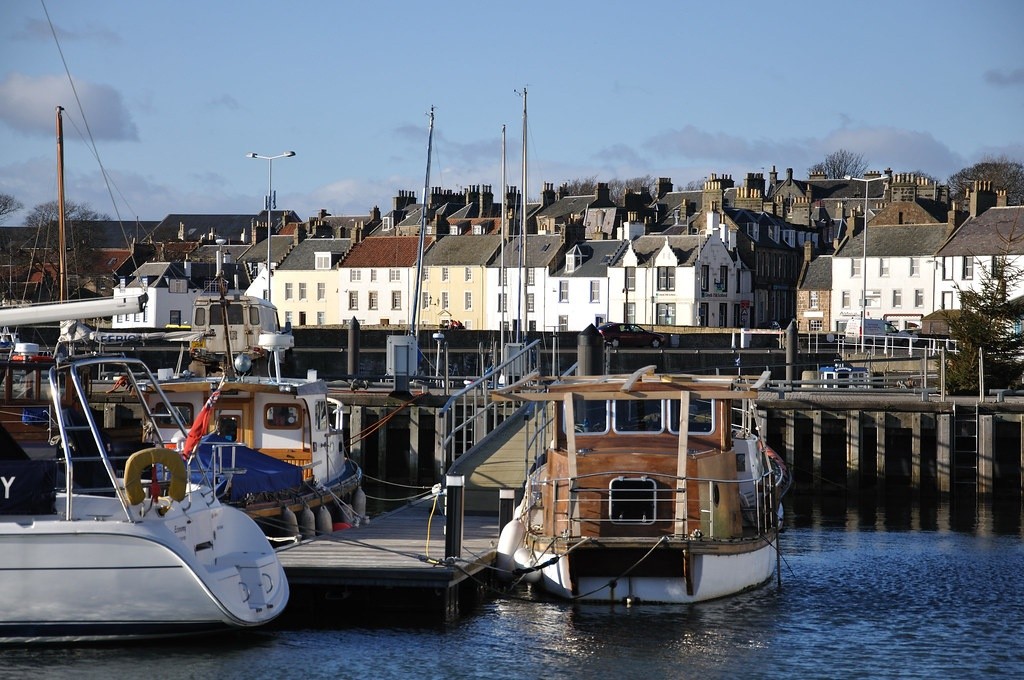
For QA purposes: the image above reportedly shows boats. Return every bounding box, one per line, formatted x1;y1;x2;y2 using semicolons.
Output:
494;379;794;607
0;356;289;646
114;334;363;549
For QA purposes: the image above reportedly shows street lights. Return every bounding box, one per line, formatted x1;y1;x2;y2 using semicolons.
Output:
244;150;296;303
843;174;890;354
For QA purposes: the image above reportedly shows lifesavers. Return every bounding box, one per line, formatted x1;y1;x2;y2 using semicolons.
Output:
124;447;187;505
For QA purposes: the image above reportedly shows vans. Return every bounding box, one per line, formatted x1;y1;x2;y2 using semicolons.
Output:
189;271;285;366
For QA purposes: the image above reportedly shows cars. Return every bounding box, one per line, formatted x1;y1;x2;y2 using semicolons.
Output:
596;322;665;350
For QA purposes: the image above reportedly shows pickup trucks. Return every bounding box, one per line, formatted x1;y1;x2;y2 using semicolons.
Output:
844;318;917;349
